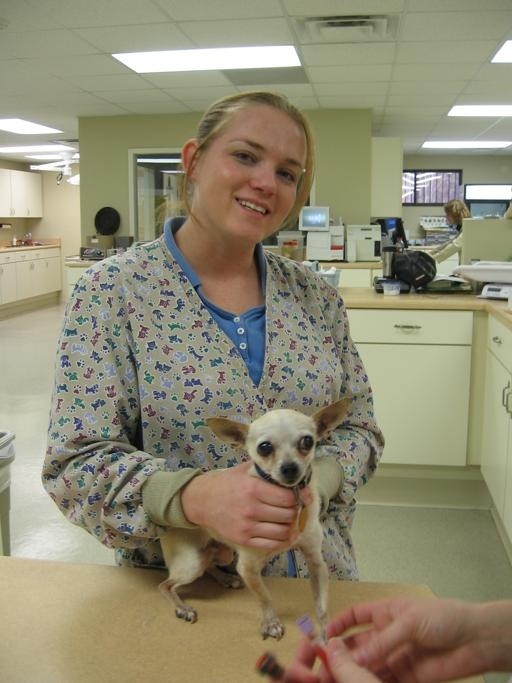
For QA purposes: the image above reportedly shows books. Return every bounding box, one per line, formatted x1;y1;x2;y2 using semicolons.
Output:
415;286;472;294
425;279;451;288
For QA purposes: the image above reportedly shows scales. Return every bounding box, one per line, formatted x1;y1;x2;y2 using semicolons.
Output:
453;261;512;301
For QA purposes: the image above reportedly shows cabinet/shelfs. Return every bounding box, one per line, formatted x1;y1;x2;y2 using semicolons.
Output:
318;259;384;289
478;313;512;565
345;307;474;472
0;248;62;306
65;260;103;299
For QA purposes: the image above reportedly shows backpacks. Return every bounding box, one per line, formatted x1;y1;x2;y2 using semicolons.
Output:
392;251;436;286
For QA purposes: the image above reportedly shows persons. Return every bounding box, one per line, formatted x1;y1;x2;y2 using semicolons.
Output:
425;198;472;266
274;594;512;683
40;88;388;586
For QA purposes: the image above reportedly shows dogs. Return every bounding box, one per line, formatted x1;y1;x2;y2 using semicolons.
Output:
157;394;354;641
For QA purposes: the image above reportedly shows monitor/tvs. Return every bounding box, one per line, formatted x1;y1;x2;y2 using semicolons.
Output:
392;218;408;248
299;206;330;231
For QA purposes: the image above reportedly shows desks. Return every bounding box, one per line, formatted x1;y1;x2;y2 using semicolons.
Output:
0;556;487;683
